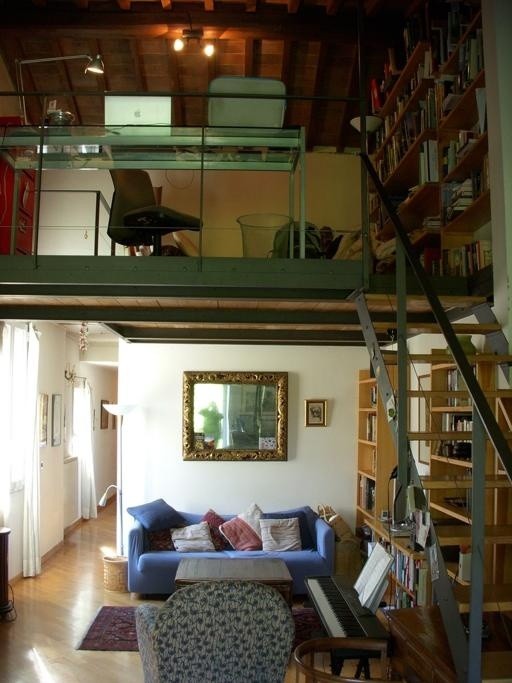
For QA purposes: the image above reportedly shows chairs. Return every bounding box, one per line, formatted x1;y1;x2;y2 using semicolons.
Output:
132;575;297;683
205;74;290;163
291;633;389;681
107;164;204;256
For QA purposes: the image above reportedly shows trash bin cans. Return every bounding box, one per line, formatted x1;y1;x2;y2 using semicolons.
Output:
103;556;128;592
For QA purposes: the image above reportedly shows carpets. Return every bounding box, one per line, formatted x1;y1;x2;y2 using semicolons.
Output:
73;604;328;653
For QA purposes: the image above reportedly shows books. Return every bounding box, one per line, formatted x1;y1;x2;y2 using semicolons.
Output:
370;2;492;279
360;367;476;608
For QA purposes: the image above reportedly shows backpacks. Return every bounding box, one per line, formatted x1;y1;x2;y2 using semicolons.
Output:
269;220;323;259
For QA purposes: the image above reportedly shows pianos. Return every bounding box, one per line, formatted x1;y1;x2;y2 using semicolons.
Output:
304;574;391;656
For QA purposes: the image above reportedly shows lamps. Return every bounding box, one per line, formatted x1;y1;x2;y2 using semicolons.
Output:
95;401;126;555
65;362;78;381
349;112;384;257
172;10;217;59
14;50;107;134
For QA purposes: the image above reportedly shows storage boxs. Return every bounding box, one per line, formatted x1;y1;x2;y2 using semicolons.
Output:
100;92;175;137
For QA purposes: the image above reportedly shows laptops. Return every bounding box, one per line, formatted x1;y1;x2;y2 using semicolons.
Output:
103;96;172;136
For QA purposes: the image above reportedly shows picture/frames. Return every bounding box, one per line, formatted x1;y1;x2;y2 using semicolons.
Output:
36;392;48;444
51;392;63;448
416;372;430;465
100;397;109;429
304;397;328;427
112;402;117;430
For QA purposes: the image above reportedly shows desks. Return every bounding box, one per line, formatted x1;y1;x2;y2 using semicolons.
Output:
0;120;312;257
381;605;511;682
175;558;294;621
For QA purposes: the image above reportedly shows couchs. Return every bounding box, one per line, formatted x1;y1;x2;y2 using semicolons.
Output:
126;497;338;602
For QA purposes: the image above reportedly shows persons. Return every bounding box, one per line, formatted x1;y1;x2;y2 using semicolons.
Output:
199;401;224;449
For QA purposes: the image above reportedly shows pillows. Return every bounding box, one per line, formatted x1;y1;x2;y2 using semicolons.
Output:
126;497;316;553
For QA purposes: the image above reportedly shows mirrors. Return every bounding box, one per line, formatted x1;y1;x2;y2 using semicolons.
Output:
182;369;289;461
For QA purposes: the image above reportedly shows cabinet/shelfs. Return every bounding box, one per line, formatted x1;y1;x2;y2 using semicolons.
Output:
352;360;412;560
428;345;511;658
355;1;492;279
0;114;41;255
0;526;18;621
